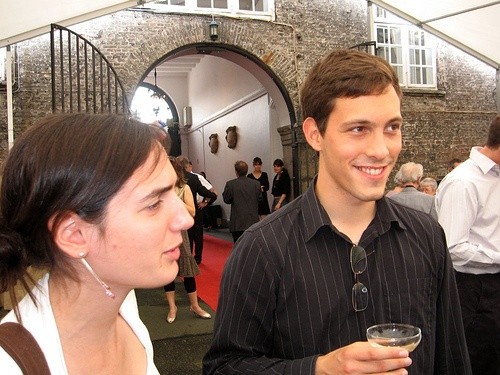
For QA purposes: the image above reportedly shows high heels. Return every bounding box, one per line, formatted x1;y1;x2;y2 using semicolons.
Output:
167;307;177;324
189;303;211;319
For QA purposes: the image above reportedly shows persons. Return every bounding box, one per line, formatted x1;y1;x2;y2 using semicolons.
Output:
0;113;194;375
247;157;271;221
222;160;262;244
271;159;290;213
164;155;223;324
202;51;476;375
434;117;500;375
385;158;462;221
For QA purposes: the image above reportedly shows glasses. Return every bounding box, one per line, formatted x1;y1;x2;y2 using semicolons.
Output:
349;243;377;313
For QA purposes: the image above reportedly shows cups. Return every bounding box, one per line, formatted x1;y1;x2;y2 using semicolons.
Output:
366;324;422;373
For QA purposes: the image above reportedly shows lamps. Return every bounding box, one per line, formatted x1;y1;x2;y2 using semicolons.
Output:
209;14;218;40
